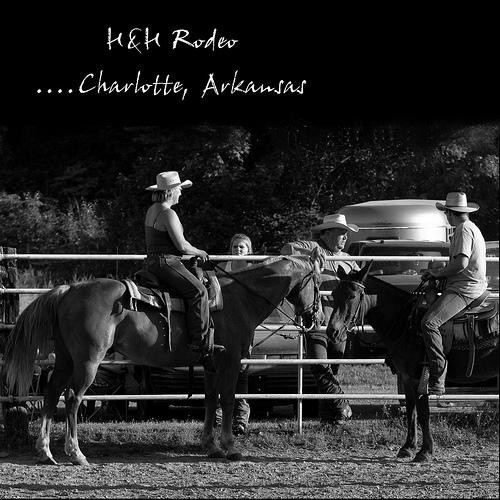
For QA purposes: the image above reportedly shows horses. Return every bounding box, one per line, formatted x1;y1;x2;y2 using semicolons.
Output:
326;257;500;466
1;246;325;467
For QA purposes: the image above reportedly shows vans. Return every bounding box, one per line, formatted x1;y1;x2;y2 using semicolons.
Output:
100;299;319;421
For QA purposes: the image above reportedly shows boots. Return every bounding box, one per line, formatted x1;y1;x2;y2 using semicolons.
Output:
232;398;251;432
317;368;352;423
215;400;223;427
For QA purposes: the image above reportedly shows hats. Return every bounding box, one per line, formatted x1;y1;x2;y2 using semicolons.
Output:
436;192;480;213
311;214;360;234
144;170;193;191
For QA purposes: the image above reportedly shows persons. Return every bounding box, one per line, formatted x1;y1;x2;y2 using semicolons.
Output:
143;170;226;357
418;192;489;396
210;233;266;432
280;214;365;426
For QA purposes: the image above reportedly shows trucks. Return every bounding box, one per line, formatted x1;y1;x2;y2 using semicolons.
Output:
336;200;500;355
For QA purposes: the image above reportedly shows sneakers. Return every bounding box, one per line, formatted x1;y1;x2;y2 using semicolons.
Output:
415;383;446;394
189;344;225;352
